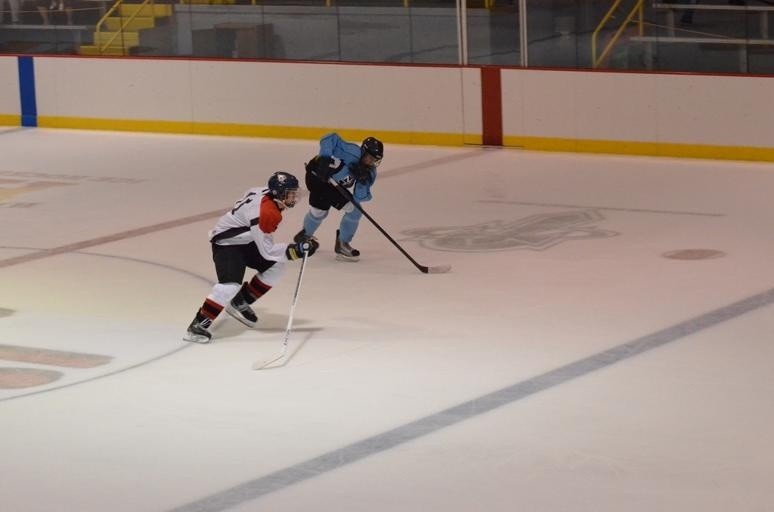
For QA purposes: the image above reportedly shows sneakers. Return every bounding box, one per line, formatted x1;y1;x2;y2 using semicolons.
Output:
231;282;258;323
187;312;212;340
335;240;361;256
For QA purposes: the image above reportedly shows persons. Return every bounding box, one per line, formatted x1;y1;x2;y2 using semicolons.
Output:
182;171;319;343
294;133;383;257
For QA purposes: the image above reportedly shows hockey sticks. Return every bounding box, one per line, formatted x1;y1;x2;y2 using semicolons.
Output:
329;176;452;274
252;250;308;369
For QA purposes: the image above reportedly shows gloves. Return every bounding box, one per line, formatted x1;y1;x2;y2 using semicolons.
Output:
319;158;332;182
286;239;320;261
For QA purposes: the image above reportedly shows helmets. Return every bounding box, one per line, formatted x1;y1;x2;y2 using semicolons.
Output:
363;136;384;159
268;171;299;189
